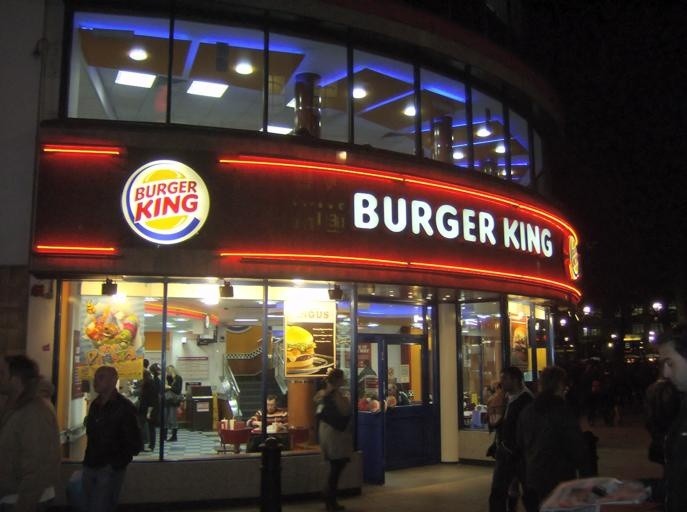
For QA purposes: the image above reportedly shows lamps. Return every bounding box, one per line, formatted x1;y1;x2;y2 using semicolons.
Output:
218;281;235;299
328;284;344;300
99;278;119;298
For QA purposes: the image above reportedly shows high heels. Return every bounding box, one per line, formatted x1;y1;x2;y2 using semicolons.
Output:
326;501;345;510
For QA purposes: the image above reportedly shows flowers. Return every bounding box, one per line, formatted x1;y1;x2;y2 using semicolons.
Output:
355;391;397;413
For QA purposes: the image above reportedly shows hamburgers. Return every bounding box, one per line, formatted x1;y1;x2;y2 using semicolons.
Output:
286;326;319;369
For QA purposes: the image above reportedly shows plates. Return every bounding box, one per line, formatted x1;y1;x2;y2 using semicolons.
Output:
286;356;329;374
561;481;648;508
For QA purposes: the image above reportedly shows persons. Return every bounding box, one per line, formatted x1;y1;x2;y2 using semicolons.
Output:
137;325;686;512
83;365;143;512
0;354;67;510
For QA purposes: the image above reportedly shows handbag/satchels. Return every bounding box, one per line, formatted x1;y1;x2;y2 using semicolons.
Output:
164;390;184;407
315;389;352;432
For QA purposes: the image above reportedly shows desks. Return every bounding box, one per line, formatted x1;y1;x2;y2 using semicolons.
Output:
218;426;255;453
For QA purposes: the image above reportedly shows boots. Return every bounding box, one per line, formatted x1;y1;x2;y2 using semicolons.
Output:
168;429;177;440
162;429;167;440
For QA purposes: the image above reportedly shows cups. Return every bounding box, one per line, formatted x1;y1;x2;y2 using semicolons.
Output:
130;380;138;395
229;419;237;431
221;420;228;431
271;422;278;434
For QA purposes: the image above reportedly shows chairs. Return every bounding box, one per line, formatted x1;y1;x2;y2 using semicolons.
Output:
243;428;291;454
